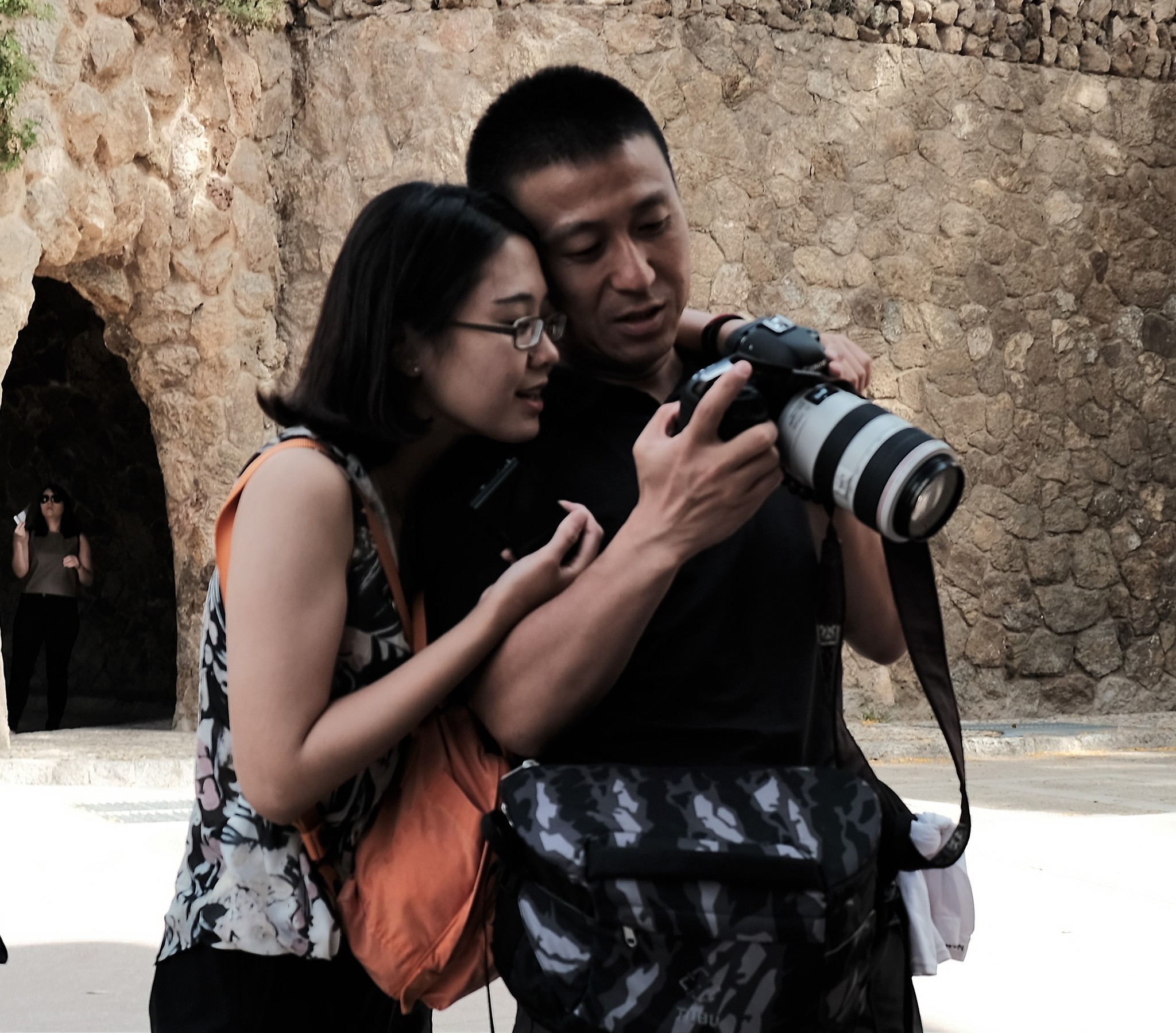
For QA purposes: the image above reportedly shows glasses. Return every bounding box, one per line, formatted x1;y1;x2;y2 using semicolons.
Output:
454;309;569;354
40;494;63;504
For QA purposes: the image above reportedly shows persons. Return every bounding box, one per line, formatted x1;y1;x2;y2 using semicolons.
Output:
5;483;94;735
145;181;870;1033
431;66;929;1033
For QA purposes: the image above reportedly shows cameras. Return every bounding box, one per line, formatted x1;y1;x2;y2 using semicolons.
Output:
671;313;966;544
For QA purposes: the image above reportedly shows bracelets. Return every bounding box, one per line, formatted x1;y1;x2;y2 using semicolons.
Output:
74;562;81;569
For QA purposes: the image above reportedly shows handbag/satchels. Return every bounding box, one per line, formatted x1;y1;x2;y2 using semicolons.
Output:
478;751;916;1032
330;706;510;1013
